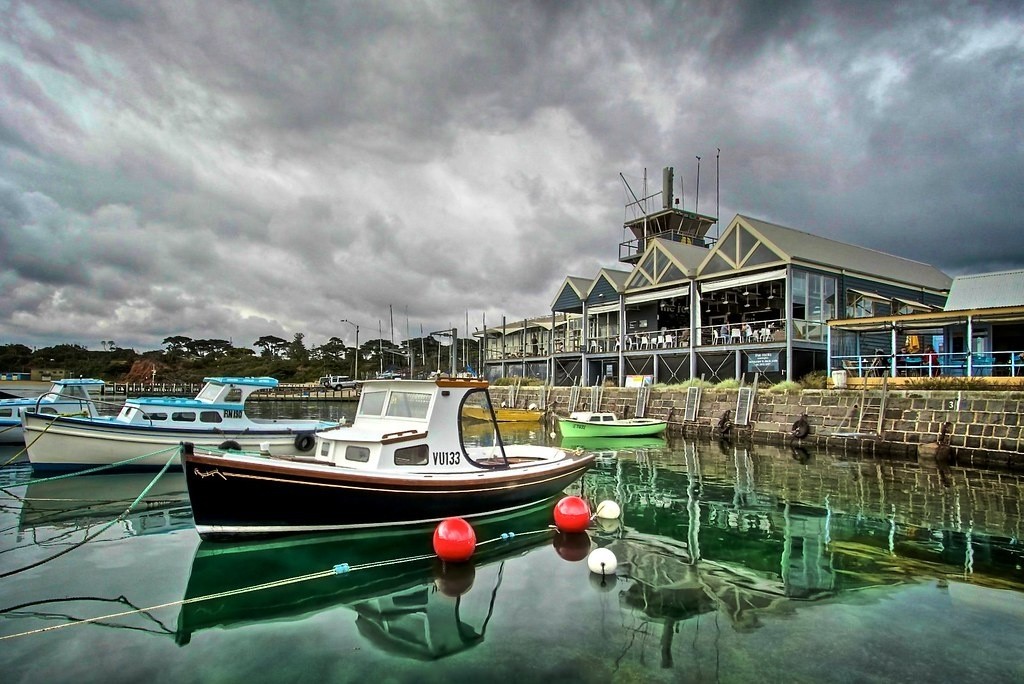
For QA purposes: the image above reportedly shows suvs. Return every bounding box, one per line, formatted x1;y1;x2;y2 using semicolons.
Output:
330;375;359;391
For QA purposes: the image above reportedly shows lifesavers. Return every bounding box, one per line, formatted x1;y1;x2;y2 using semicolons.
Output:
218;440;241;450
295;432;315;451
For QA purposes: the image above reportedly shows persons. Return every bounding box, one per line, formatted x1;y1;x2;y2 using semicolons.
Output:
721;321;730;344
741;323;752;343
896;347;913;375
877;344;941;377
531;335;538;356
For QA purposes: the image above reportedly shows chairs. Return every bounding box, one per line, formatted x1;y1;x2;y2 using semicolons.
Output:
656;336;665;349
730;328;742;344
649;338;657;349
746;330;759;343
679;337;689;347
759;327;771;342
713;330;726;345
628;337;639;350
640;337;648;350
663;335;674;348
589;340;601;354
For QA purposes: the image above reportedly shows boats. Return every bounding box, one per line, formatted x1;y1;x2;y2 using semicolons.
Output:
466;404;547;423
179;375;596;542
0;374;120;447
173;489;578;645
558;406;668;440
19;371;351;474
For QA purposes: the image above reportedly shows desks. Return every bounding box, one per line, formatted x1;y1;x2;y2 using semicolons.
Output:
598;337;615;352
903;357;922;377
850;360;873;367
730;330;743;332
951;357;996;376
768;327;780;333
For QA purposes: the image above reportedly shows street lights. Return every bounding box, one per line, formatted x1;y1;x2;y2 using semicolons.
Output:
340;319;360;381
